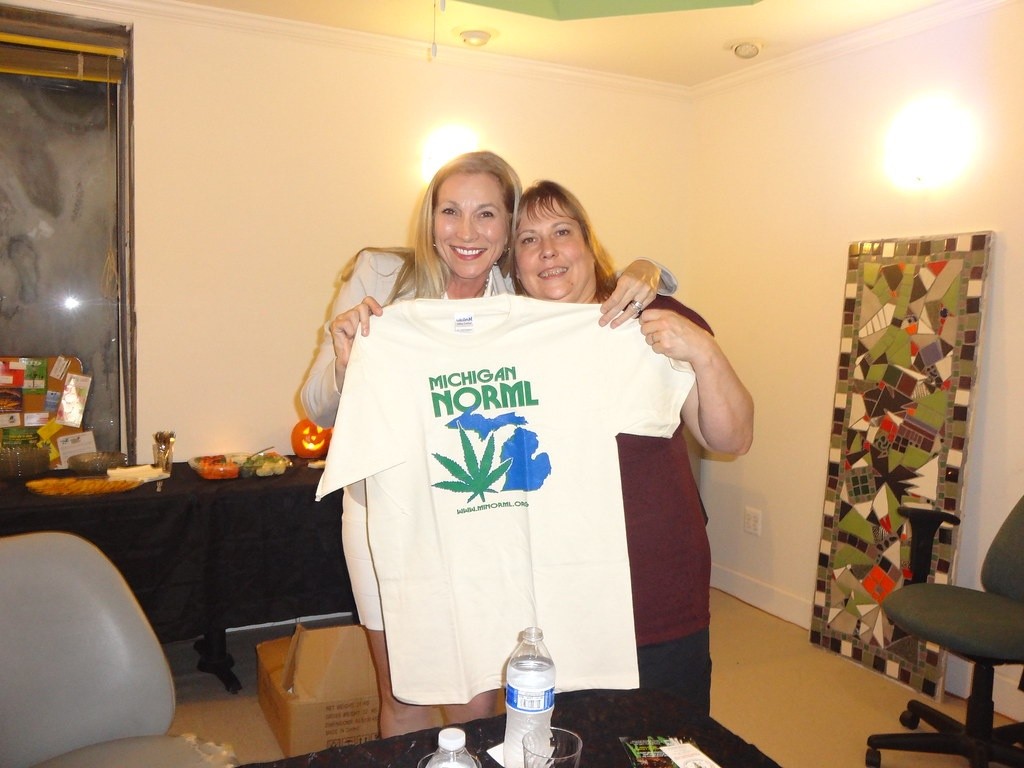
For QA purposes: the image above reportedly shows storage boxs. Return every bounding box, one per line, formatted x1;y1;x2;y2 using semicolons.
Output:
255;622;382;758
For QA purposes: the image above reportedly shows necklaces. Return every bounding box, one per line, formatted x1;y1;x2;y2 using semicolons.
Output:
483;277;490;289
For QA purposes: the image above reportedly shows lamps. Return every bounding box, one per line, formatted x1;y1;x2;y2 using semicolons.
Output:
459;31;490;46
724;39;768;60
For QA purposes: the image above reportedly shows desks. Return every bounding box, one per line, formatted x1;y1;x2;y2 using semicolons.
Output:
237;684;784;768
0;453;359;693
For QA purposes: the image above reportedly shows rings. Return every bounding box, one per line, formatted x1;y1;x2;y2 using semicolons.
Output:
631;301;642;314
651;333;657;343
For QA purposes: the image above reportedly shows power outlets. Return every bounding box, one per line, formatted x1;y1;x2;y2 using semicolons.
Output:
744;507;762;536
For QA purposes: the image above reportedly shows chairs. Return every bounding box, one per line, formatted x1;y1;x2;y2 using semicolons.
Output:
866;494;1024;768
0;532;242;768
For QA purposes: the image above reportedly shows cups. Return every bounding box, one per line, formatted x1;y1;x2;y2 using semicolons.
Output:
522;729;583;768
153;444;173;473
417;753;482;768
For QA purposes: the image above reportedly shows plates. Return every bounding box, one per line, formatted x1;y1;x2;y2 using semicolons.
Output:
68;451;127;472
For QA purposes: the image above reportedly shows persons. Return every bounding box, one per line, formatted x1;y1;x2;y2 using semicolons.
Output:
300;151;678;738
514;181;756;714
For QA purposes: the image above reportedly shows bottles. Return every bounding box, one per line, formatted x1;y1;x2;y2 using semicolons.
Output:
503;626;553;768
425;727;477;768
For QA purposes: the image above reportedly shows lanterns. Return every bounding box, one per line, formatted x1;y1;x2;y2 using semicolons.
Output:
292;419;332;458
